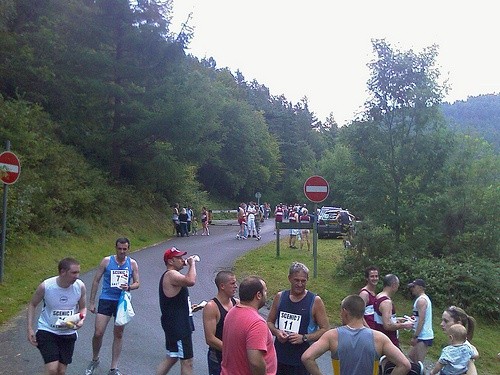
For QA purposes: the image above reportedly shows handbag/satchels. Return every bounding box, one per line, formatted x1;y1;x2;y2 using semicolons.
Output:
115;290;136;326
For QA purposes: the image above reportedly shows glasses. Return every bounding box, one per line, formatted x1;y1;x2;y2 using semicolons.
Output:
449;306;461;321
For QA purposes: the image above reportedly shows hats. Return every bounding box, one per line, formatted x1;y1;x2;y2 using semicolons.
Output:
164;247;187;261
408;279;426;287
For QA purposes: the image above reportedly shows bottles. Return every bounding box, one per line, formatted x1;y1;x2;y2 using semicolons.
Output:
183;255;200;266
64;313;84;323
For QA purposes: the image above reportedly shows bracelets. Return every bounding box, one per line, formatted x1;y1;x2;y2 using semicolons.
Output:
127;285;130;291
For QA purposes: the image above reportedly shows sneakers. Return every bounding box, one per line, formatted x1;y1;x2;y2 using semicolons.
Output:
84;357;100;375
107;368;124;375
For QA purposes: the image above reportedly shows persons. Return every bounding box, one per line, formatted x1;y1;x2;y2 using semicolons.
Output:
157;246;199;375
374;274;413;346
201;207;210;236
221;275;278;375
336;210;356;250
85;237;140;375
236;201;271;240
357;267;378;330
273;202;308;240
440;305;479;375
267;261;330;375
408;280;435;375
202;271;240;375
288;207;299;249
299;210;311;251
172;202;198;237
301;294;412;375
27;258;87;375
430;324;479;375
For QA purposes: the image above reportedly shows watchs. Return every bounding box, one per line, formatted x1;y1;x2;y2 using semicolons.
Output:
302;334;308;343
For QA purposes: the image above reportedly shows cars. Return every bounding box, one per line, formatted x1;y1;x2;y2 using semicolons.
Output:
316;206;342;233
317;209;355;239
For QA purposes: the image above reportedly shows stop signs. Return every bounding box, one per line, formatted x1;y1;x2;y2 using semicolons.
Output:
304;175;330;203
0;150;21;185
